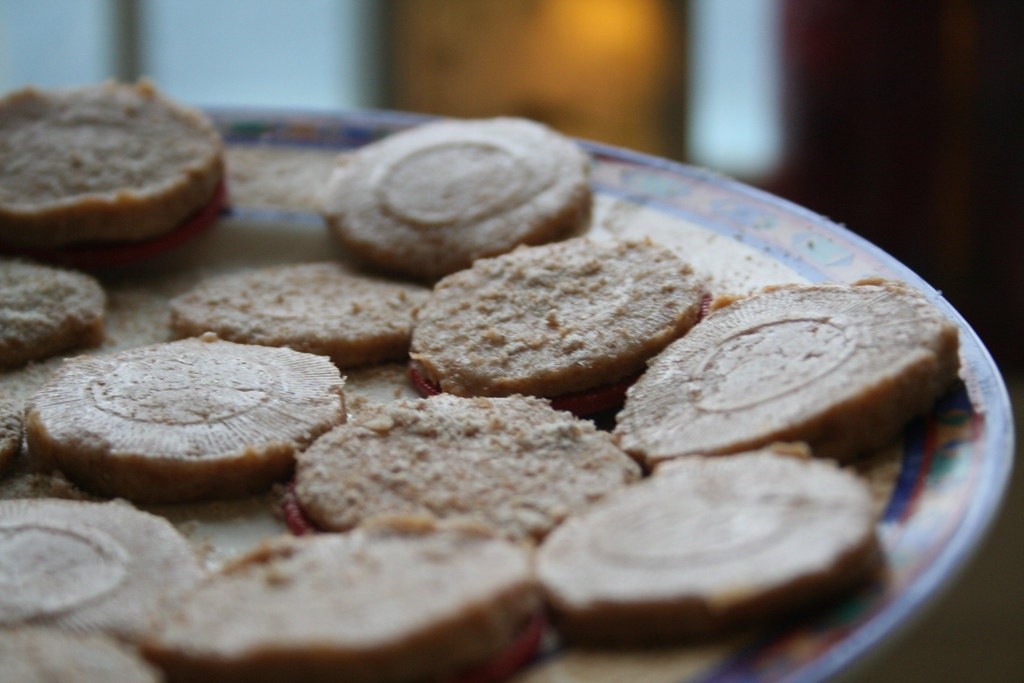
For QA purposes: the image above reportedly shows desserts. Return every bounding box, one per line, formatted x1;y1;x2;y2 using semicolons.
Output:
0;84;963;683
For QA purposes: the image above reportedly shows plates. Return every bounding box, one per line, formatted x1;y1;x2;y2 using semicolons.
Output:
0;100;1016;683
